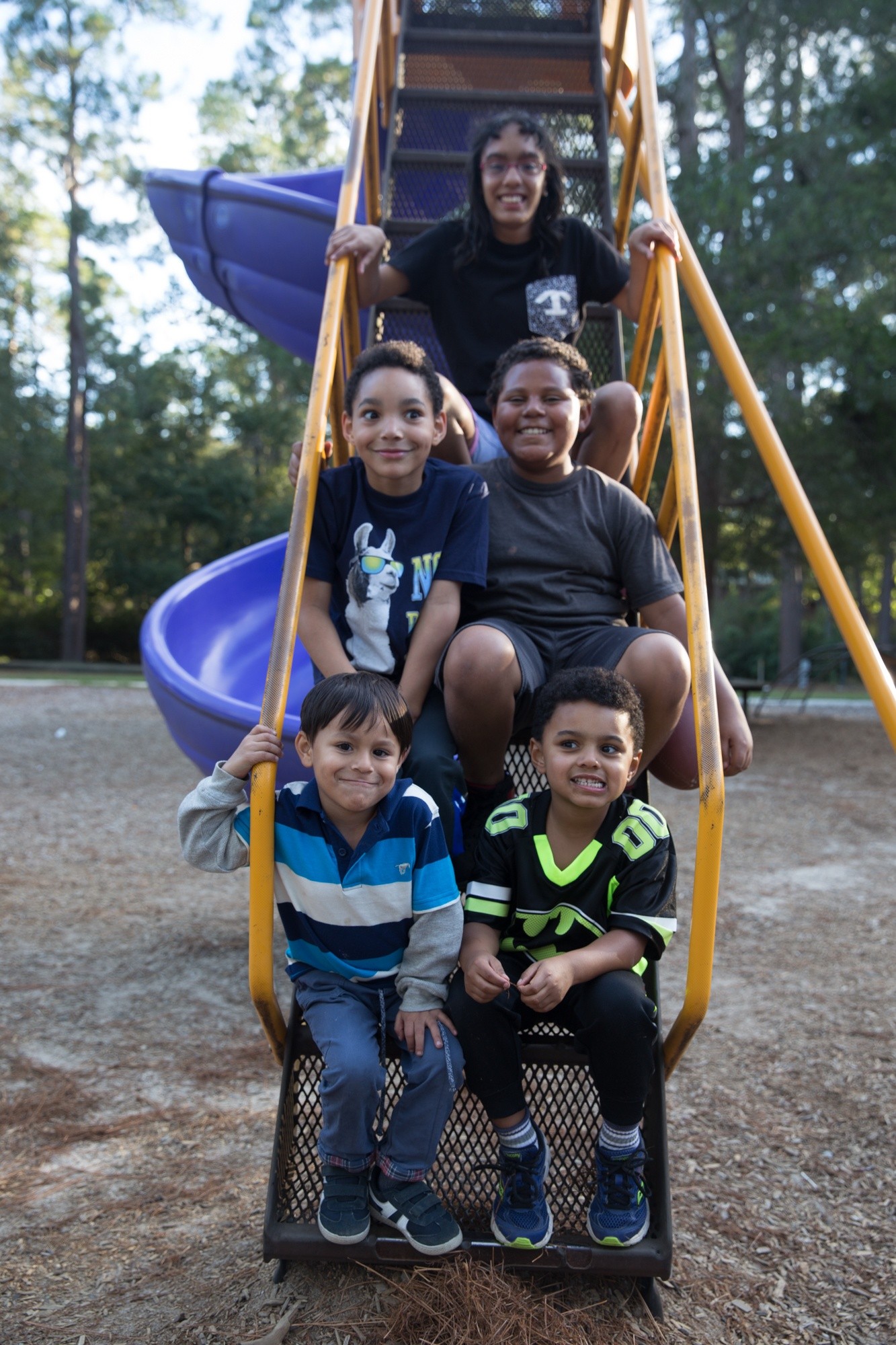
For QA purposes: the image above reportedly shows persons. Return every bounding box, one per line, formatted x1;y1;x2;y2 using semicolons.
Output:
177;672;465;1256
318;111;682;481
288;337;754;812
295;342;488;848
444;666;677;1247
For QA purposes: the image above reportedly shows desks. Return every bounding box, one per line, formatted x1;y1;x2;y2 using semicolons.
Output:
732;677;767;720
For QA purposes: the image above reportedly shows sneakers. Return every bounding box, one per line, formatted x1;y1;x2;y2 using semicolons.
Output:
318;1163;371;1245
474;1112;554;1250
368;1164;463;1255
587;1126;651;1248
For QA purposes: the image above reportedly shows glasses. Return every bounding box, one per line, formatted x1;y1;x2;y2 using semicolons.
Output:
480;158;549;176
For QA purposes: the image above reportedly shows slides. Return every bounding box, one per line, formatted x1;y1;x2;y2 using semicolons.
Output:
141;161;516;867
